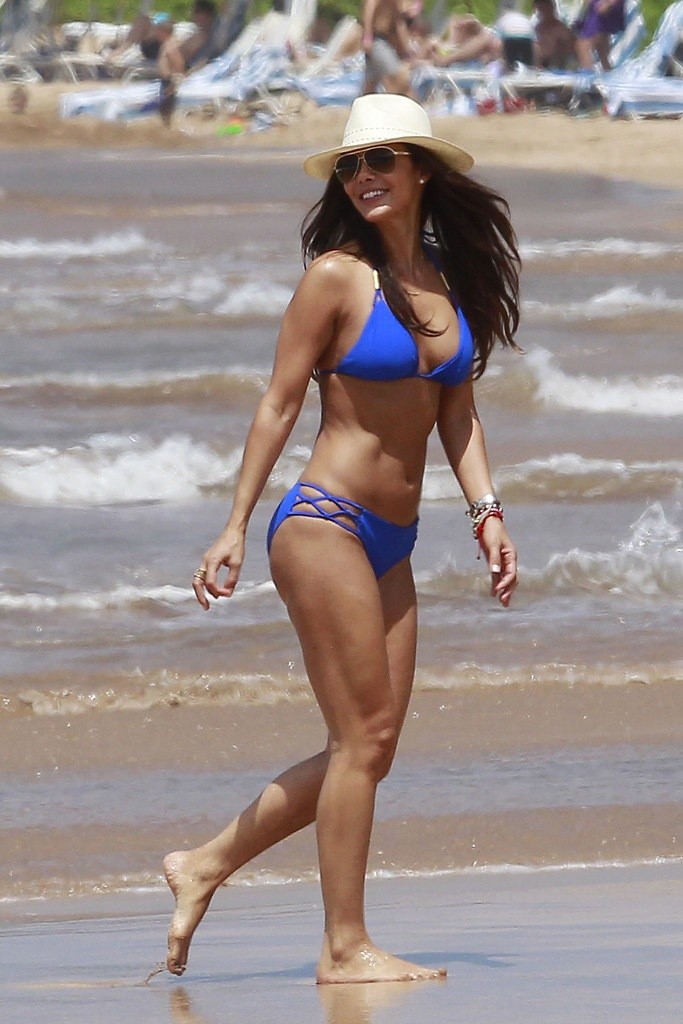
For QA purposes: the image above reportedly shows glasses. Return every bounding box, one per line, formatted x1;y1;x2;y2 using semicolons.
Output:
333;146;412;183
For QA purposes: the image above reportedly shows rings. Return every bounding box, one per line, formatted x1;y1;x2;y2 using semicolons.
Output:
194;568;206;582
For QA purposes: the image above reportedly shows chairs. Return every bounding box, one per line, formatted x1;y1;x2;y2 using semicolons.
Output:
58;0;683;122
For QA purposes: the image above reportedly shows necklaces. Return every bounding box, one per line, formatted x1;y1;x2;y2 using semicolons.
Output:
386;246;426;296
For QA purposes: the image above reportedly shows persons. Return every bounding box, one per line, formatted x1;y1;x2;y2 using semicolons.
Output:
162;93;522;984
0;0;683;129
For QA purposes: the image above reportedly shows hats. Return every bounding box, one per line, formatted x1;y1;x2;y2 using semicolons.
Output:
303;94;475;183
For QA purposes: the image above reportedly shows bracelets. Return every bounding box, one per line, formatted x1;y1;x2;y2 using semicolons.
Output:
465;494;504;560
